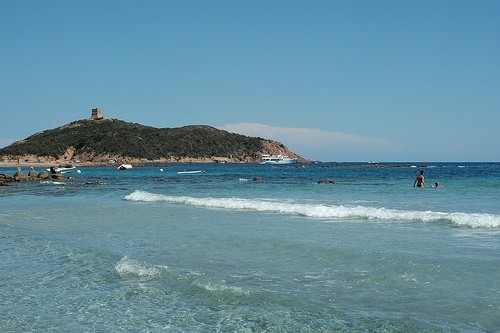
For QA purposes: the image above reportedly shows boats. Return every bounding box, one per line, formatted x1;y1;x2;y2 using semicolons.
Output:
117;163;133;169
46;163;76;171
262;154;296;164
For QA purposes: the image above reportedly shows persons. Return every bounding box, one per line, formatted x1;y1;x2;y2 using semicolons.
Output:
413;170;426;188
50;167;57;173
434;182;444;187
